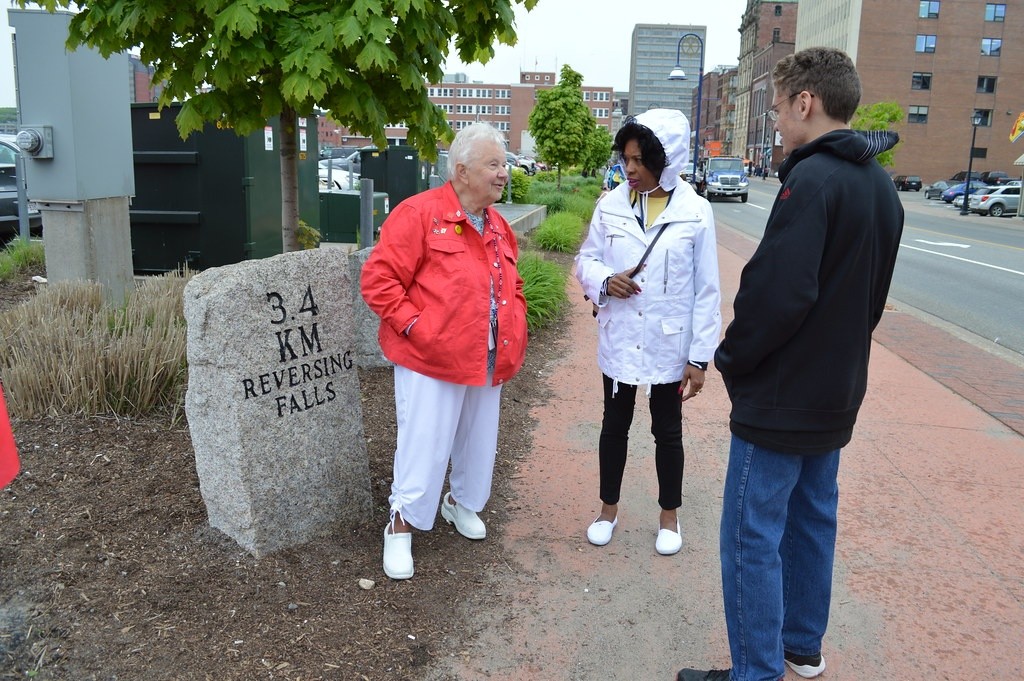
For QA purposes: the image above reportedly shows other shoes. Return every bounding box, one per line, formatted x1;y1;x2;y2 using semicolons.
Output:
587;512;617;545
655;516;682;554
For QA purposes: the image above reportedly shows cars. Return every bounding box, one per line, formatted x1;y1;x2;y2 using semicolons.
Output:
1;123;537;255
922;168;1023;216
894;175;923;192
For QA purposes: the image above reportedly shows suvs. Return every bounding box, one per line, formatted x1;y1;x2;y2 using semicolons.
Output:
698;152;750;204
507;152;539;179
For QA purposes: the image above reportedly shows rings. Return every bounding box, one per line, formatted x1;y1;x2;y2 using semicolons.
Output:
695;391;699;395
699;389;702;392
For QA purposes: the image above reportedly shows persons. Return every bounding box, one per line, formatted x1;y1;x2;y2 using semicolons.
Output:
573;108;722;556
679;48;904;681
608;165;626;191
360;122;527;579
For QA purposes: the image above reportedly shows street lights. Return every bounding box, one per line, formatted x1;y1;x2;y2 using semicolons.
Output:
666;28;705;186
961;112;983;216
762;134;770;181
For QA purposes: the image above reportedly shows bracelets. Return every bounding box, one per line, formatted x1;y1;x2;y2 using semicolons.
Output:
687;360;708;372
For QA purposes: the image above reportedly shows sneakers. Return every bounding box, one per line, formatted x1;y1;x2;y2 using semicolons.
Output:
676;667;784;681
784;650;826;678
383;521;414;579
441;492;487;539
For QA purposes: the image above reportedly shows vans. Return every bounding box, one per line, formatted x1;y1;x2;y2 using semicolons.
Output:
680;160;701;184
